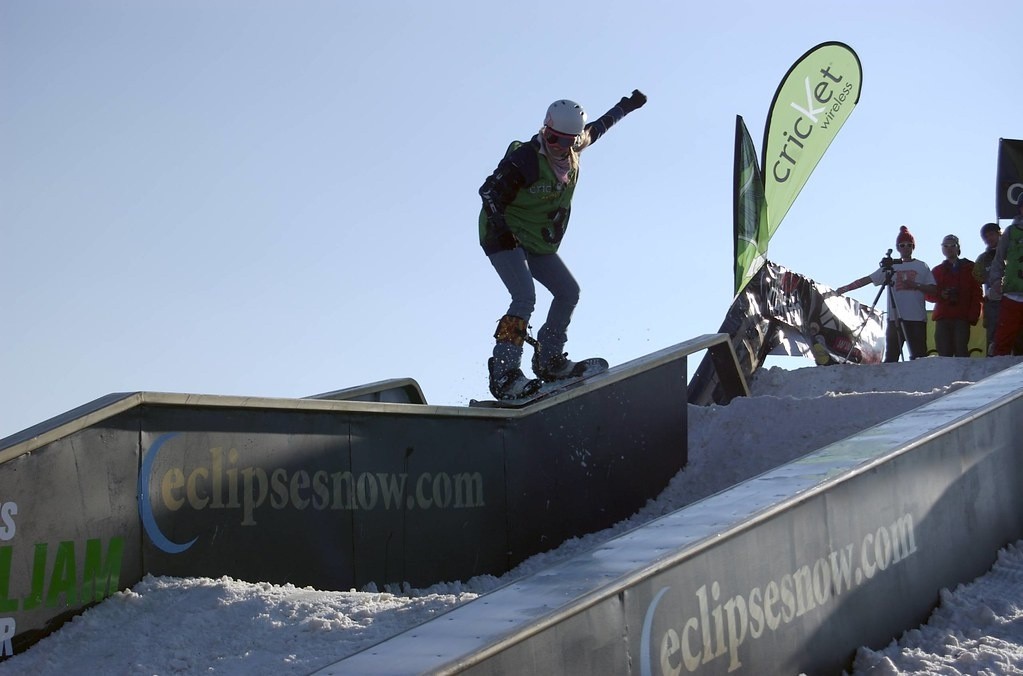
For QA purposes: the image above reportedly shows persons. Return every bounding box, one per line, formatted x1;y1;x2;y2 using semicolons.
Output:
925;234;984;357
989;193;1023;356
972;223;1007;357
835;225;937;363
478;89;647;399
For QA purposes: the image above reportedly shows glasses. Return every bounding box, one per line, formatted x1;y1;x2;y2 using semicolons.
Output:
898;243;913;248
941;243;957;248
543;127;576;148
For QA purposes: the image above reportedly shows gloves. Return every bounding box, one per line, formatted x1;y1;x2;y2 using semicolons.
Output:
629;89;647;109
498;231;521;251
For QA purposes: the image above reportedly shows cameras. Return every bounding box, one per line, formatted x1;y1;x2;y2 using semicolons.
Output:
947;287;959;304
881;249;903;267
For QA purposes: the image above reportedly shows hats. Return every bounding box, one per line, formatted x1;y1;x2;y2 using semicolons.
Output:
896;226;916;250
980;223;1001;236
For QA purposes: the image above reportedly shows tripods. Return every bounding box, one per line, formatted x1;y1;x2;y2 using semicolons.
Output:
842;267;914;364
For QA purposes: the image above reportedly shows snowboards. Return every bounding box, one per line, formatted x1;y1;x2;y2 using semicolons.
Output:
468;357;609;408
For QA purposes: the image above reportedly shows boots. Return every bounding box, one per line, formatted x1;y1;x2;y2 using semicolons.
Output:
488;341;539;398
531;325;578;377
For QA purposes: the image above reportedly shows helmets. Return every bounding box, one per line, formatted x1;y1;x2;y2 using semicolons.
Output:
543;100;587;135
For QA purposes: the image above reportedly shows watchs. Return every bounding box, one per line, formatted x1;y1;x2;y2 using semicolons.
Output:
917;283;920;289
